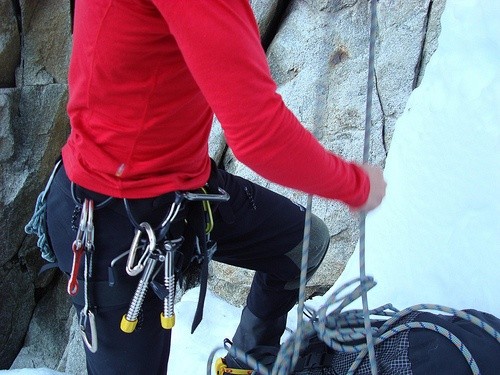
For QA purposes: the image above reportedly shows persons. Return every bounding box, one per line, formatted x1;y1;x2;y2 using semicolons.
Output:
44;0;387;375
